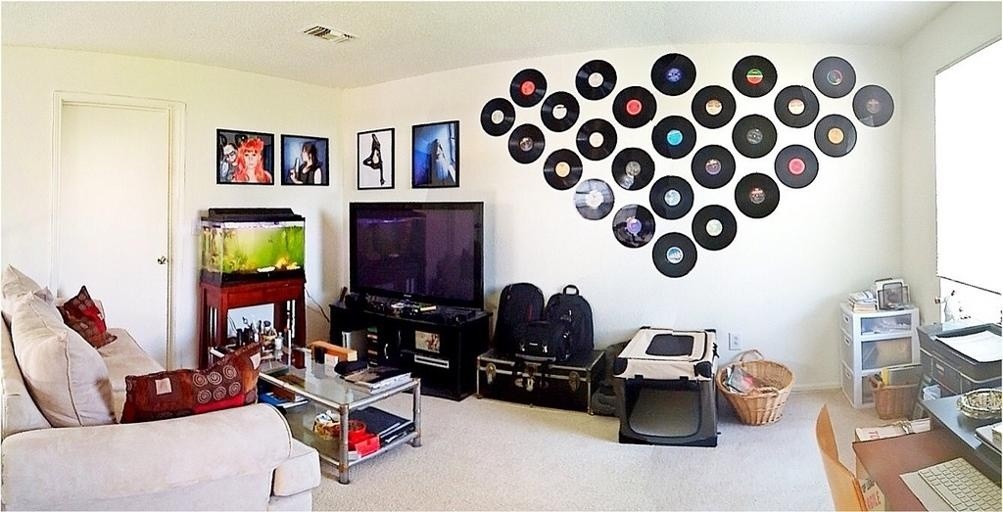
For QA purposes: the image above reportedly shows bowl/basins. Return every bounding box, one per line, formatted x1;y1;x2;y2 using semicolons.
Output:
347;420;366;441
956;388;1000;420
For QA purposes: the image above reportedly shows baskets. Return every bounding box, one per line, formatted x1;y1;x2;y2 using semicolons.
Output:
869;374;922;420
715;349;797;426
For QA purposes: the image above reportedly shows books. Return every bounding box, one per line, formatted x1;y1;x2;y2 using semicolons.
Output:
847;289;878;311
271;374;312;403
260;390;308;410
346;406;413;444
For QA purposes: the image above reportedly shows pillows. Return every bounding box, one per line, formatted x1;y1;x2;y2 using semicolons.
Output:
55;284;117;350
7;290;119;428
120;337;264;426
1;261;48;326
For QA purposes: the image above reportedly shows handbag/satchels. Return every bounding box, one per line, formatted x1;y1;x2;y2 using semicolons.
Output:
507;320;577;364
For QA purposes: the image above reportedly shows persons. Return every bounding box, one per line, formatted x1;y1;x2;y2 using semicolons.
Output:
232;137;274;183
288;142;322;186
221;141;238;183
419;137;456;186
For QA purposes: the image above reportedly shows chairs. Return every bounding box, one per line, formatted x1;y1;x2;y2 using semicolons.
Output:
808;403;872;512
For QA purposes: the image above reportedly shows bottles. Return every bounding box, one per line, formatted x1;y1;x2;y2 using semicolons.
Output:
274;332;283;351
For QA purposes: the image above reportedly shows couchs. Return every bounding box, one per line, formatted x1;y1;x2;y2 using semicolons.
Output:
1;275;324;511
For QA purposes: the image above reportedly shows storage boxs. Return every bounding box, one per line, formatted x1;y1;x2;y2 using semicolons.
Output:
854;416;930;512
473;339;608;418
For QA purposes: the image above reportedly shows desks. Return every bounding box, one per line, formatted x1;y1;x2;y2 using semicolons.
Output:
848;383;1002;511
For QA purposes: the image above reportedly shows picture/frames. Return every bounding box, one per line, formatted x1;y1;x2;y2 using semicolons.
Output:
214;127;276;188
279;132;330;187
356;127;397;190
409;119;460;190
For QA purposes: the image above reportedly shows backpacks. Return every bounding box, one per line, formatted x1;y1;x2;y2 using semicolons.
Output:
493;283;545;362
543;284;594;358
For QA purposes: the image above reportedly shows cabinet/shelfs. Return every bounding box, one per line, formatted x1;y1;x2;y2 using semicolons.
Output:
836;296;920;411
327;292;492;403
914;315;1001;398
197;274;305;382
